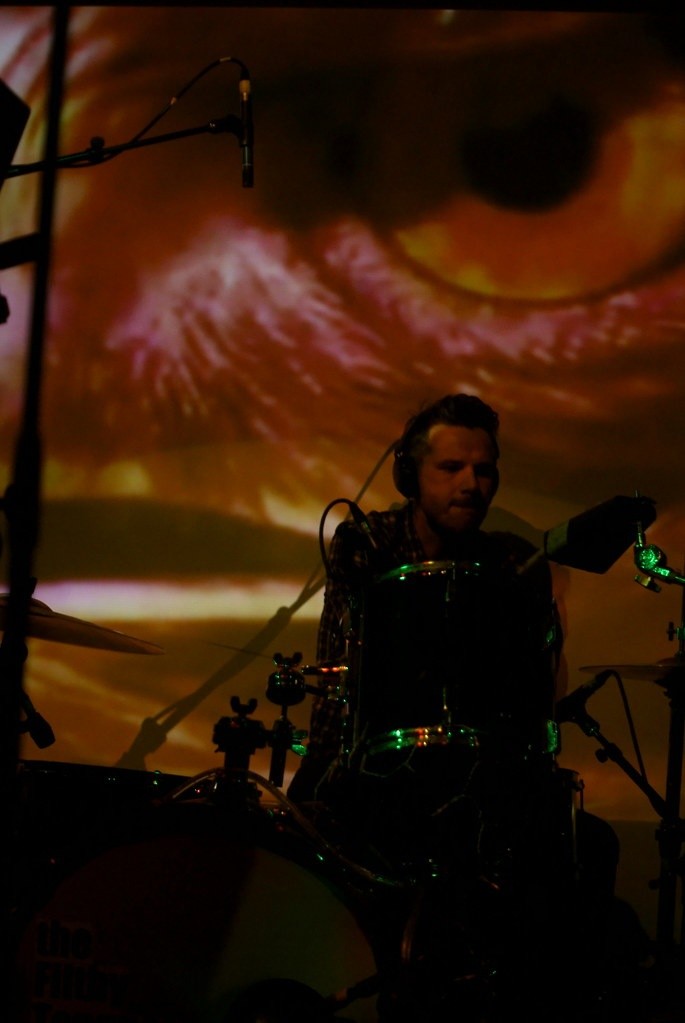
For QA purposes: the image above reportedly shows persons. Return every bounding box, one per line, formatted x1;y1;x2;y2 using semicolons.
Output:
288;393;564;802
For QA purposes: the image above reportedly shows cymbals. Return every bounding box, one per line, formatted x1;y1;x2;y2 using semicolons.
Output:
2;599;167;655
578;662;673;682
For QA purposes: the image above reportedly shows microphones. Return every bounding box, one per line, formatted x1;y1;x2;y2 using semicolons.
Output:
346;502;391;578
543;667;613;726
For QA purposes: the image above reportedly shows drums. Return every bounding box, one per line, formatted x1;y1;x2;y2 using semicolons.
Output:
319;725;586;1023
347;558;546;783
3;803;386;1022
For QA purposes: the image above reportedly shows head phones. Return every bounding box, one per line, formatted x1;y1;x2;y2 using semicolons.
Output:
392;415;500;500
